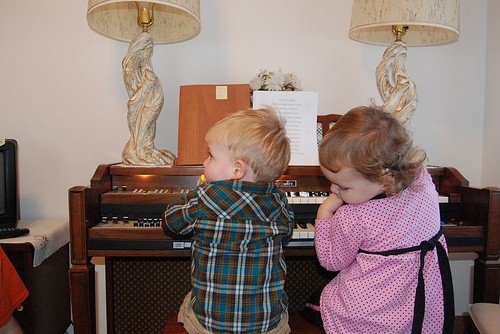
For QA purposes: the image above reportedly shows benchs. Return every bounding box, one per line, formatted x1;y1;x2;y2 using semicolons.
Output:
164;313;327;334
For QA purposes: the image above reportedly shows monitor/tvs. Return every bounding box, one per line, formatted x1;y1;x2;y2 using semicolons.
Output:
0;139;20;230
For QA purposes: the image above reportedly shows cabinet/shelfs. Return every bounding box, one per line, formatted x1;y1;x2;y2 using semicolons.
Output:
0;219;71;334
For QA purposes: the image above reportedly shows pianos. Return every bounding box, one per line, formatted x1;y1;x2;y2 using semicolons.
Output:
68;163;500;334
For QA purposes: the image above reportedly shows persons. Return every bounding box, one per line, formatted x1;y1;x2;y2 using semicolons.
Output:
313;105;455;334
0;247;30;334
161;105;297;334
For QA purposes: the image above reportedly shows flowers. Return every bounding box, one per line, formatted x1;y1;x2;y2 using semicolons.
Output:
248;69;304;91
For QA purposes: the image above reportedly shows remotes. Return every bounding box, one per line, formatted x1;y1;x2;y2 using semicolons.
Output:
0;228;30;239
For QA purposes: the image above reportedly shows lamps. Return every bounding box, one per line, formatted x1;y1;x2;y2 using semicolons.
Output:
86;0;202;168
349;0;460;126
